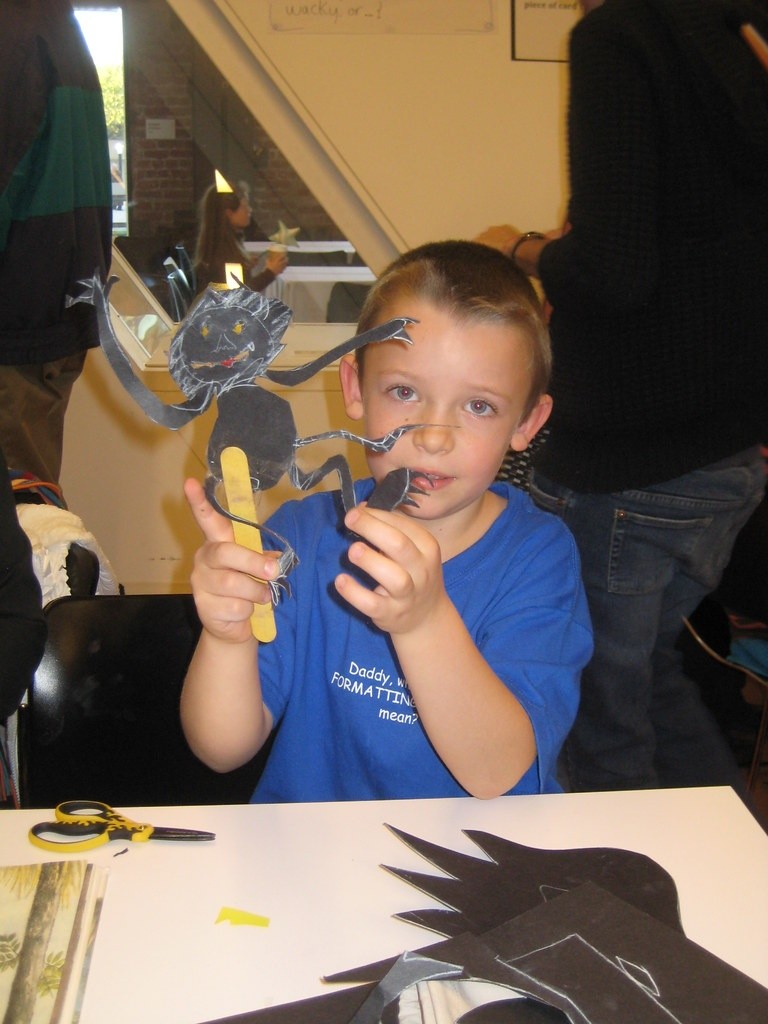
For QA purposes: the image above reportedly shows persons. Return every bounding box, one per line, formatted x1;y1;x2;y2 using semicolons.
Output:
180;241;594;805
0;0;114;499
193;179;288;299
477;0;768;793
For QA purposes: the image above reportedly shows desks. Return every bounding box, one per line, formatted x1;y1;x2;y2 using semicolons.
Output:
0;786;768;1024
264;267;380;322
244;240;367;267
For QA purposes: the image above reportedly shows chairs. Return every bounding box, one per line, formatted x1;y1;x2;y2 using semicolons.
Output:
163;245;194;323
18;591;279;809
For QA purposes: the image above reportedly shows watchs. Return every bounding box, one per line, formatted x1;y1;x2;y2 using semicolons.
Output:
502;232;547;263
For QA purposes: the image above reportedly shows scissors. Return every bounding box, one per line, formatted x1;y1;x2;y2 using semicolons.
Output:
29;797;220;855
